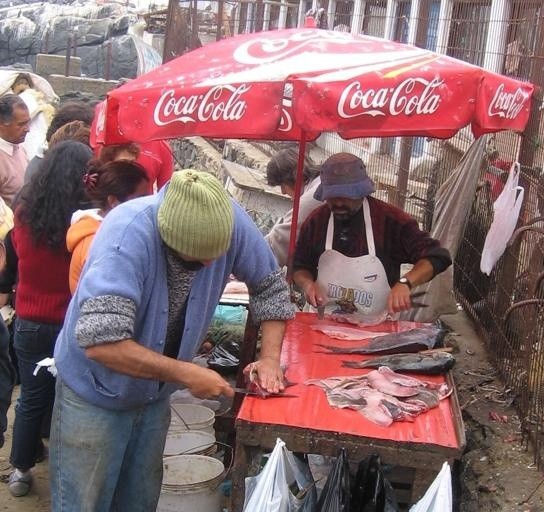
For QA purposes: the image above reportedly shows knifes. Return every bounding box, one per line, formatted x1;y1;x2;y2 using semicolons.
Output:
228;386;296;398
315;300;327;321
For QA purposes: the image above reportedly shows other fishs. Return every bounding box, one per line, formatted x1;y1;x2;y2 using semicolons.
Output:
309;322;451;356
239;361;299;400
339;350;458;376
328;290;430;329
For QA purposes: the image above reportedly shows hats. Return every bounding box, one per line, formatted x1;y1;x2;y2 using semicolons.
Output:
313;151;376;203
157;168;234;258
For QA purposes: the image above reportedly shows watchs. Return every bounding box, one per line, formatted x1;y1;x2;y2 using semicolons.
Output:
397;275;413;289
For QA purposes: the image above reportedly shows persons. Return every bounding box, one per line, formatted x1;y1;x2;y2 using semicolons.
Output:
24;103;94;181
287;149;453;326
0;94;31;447
8;142;90;497
262;144;330;269
67;99;173;296
47;166;294;512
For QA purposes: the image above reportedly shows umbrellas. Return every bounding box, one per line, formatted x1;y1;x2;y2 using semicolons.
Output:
89;11;534;296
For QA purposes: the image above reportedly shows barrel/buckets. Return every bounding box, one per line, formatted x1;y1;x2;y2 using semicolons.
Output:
155;404;233;512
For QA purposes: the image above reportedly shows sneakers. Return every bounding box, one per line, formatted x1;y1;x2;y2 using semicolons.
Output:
9;470;31;497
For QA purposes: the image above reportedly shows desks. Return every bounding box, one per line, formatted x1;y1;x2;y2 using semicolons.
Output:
228;310;466;511
